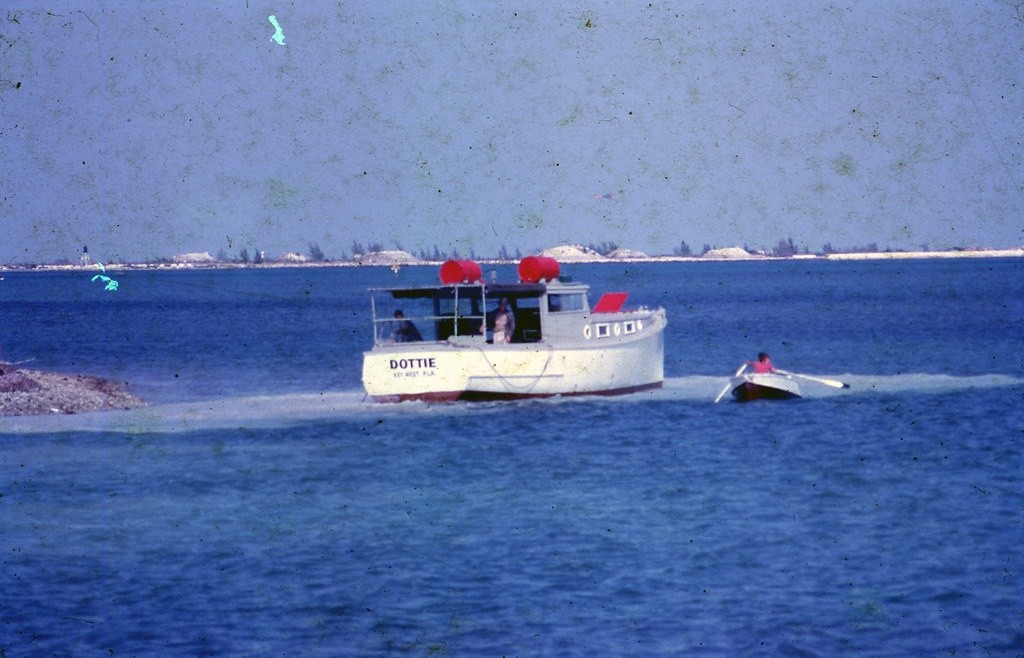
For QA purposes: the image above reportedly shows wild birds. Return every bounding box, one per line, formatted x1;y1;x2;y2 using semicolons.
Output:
267;14;287;46
91;262;119;291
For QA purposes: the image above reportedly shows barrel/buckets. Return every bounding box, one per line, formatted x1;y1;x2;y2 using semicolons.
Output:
439;259;482;286
517;256;560;284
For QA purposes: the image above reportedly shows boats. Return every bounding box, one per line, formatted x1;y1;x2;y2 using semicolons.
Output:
360;255;668;401
728;373;803;402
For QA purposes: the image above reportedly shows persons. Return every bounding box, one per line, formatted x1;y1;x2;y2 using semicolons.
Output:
746;352;780;374
479;297;516;344
393;309;424;343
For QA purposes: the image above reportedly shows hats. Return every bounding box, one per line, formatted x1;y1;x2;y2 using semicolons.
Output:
498;297;508;305
394;310;404;317
